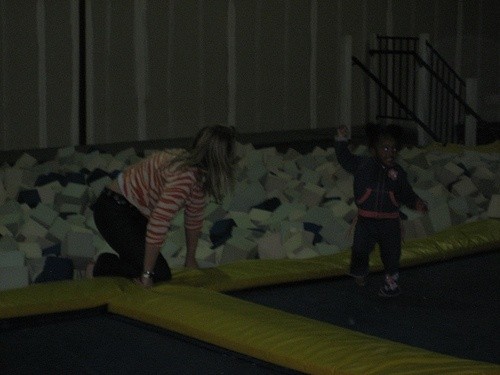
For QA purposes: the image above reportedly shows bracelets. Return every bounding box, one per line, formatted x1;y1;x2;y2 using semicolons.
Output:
140;267;159;279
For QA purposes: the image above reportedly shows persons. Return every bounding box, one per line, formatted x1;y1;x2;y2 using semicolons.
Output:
332;121;426;295
83;124;233;289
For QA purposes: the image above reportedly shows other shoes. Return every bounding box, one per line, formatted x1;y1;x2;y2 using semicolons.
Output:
353;272;369;287
379;272;401;297
